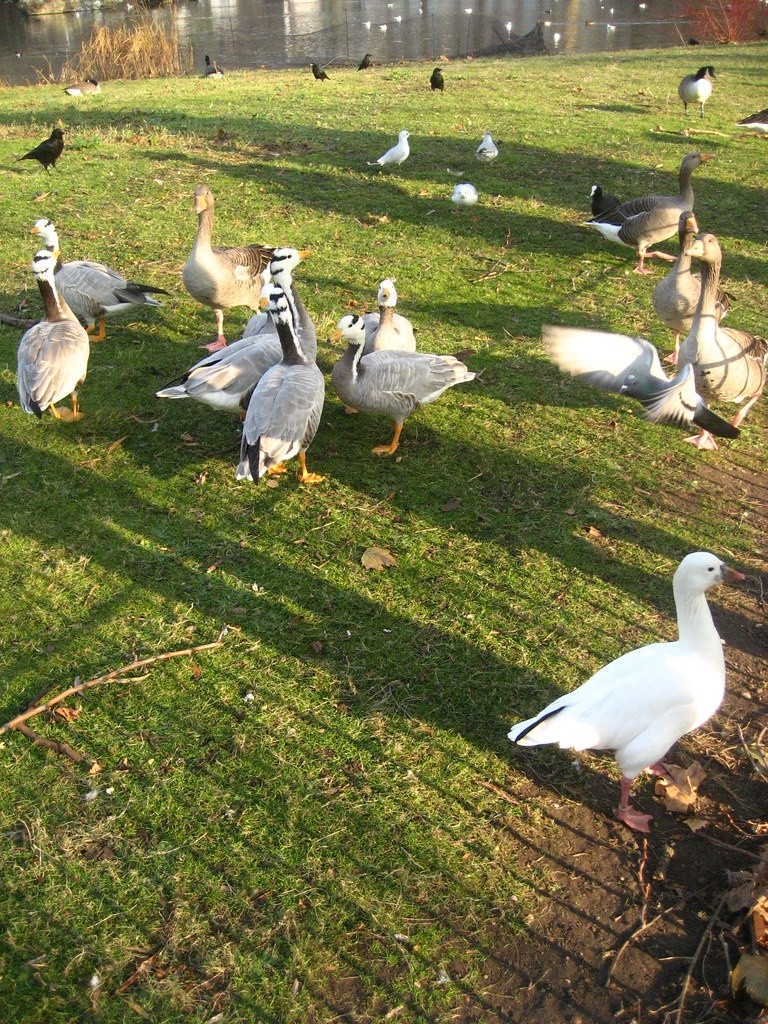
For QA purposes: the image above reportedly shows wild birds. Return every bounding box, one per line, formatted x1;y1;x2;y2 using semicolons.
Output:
309;61;331;82
589;185;621;215
357;53;373;72
541;324;743;440
430;68;444;92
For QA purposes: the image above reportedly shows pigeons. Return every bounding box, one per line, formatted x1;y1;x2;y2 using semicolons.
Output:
16;128;65;177
366;130;413;170
450;180;479;210
476;131;499;168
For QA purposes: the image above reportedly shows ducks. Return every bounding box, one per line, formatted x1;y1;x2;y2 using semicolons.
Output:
679;65;768;136
581;152;714;275
651;212;766;452
361;0;647;41
61;78;101;97
505;550;745;835
326;280;485;456
182;183;282;353
15;218;173;424
154;246;330;486
204;54;224;80
